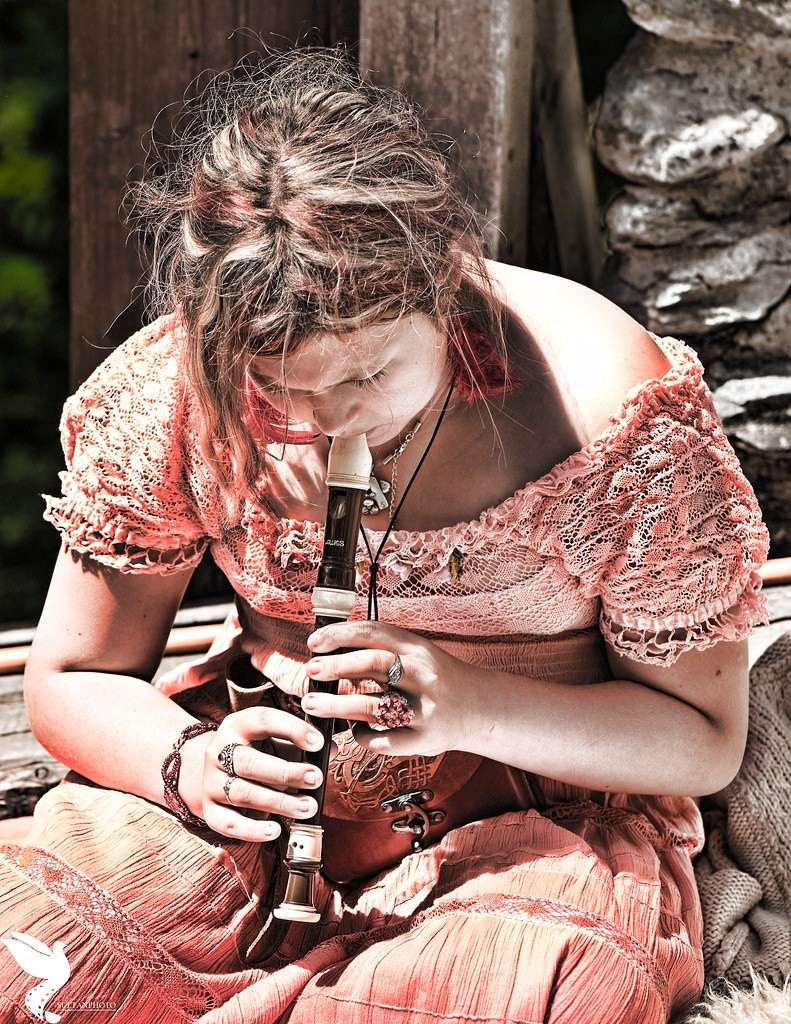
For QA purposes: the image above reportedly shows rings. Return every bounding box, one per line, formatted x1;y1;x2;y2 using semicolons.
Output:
372;689;415;731
224;775;240;807
383;653;404;685
216;742;241;774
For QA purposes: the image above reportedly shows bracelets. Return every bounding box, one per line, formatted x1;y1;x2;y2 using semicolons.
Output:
161;723;217;829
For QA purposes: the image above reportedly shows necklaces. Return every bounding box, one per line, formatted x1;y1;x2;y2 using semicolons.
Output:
324;367;460;527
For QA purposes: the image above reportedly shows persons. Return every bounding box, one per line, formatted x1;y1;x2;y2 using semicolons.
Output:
0;37;768;1022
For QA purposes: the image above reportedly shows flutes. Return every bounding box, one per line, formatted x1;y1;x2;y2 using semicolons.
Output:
274;425;373;926
